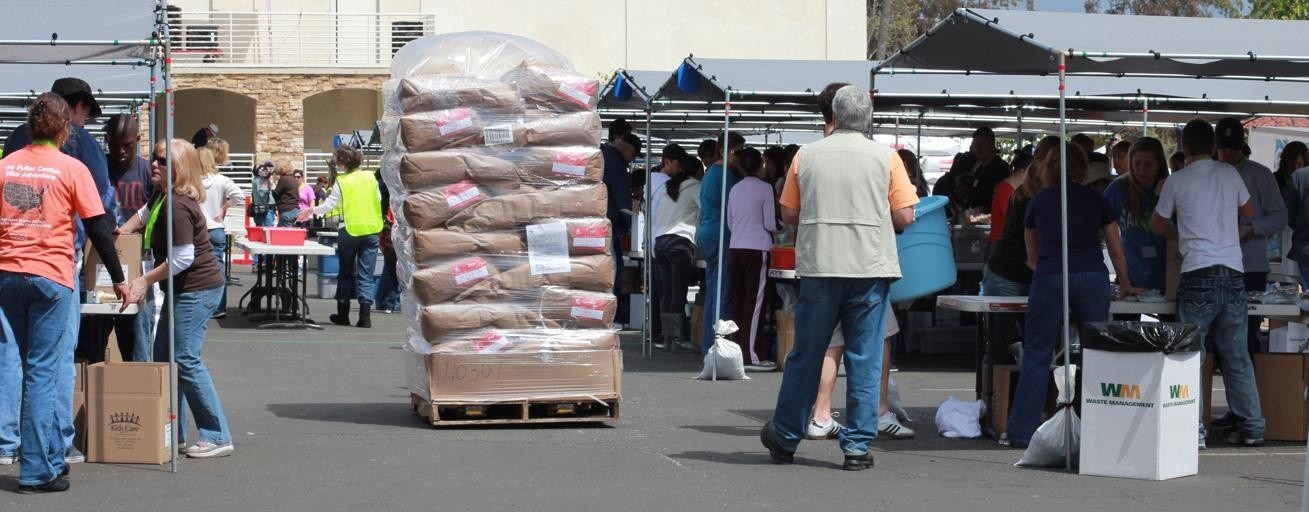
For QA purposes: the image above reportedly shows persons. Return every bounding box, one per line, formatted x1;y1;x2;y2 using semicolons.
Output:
196;138;242;317
892;117;1309;448
297;143;401;328
252;161;339;272
0;91;132;492
603;118;802;372
74;111;160;361
0;77;124;464
116;137;233;458
760;82;915;470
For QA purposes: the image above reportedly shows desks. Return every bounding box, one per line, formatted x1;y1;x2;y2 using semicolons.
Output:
232;240;336;330
222;229;247;288
317;230;339;238
75;303;139;362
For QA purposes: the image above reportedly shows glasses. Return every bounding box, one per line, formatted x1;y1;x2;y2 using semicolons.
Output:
153;153;166;167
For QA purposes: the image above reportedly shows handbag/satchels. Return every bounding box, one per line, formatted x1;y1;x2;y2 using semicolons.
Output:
249;203;270;217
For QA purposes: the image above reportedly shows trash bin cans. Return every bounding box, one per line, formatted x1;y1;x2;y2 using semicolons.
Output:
1079;322;1203;482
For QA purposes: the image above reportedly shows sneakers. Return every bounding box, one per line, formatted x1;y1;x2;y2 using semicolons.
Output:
19;448;85;496
1225;432;1262;446
211;309;226;318
1199;440;1207;449
1;454;20;466
1000;431;1010;447
807;417;846;439
745;360;777;373
761;420;793;464
877;412;914;439
844;448;875;468
181;442;234;460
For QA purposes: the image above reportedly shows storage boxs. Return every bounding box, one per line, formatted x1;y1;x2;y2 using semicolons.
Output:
260;227;306;247
245;226;263;242
83;234;142;305
86;361;179;463
629;211;1308;480
73;362;86;457
408;348;622;403
317;250;385;300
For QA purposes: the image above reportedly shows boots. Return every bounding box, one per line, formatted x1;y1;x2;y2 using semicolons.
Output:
357;304;372;327
330;303;350;326
659;313;698;354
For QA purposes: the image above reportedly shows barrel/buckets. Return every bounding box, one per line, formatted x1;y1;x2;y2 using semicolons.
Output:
889;195;957;308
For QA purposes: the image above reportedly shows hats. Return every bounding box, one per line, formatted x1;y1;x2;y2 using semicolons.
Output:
1216;117;1243;149
1082;161;1115;186
52;78;103;117
625;134;646;159
207;123;218;135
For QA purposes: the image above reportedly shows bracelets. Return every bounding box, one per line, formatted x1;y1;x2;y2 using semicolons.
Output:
113;280;125;285
